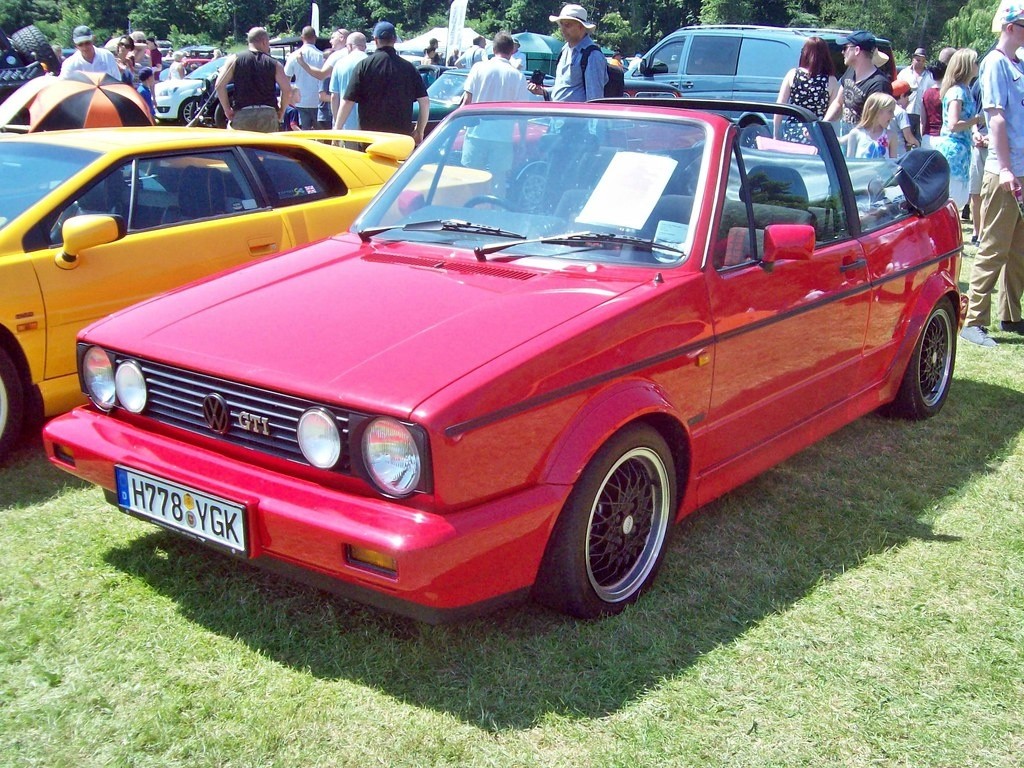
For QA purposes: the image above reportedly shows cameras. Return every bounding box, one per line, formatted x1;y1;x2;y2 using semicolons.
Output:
530;69;545;86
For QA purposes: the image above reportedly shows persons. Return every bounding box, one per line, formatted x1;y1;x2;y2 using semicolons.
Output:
846;93;899;160
627;52;642;70
823;27;894;122
422;38;445;67
608;51;623;70
281;28;371;152
113;27;222;126
526;4;609;149
670;55;677;61
456;30;529;212
52;44;66;79
891;0;1024;345
56;25;121;82
771;36;843;148
448;48;459;66
334;21;430;160
216;25;292;131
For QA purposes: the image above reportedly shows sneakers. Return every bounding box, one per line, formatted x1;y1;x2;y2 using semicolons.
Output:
959;324;997;347
1000;318;1024;334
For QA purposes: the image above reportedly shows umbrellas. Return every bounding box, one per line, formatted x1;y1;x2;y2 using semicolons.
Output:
28;68;157;133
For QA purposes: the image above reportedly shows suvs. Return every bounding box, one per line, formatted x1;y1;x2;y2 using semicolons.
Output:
621;21;897;148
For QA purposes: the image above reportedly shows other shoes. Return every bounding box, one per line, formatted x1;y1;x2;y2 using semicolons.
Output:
971;236;978;243
961;204;971;220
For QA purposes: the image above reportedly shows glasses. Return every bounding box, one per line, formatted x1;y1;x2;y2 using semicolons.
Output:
137;40;146;43
78;42;91;47
843;45;862;52
335;27;342;34
560;21;578;28
973;61;979;66
118;42;132;49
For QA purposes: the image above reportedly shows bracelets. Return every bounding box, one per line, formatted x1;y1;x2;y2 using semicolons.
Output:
1000;169;1010;173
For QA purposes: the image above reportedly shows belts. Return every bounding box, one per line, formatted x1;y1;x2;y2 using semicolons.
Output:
241;105;275;109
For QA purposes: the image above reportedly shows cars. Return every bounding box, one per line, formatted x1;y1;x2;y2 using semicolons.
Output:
151;37;696;214
42;93;970;623
60;40;225;84
0;125;495;464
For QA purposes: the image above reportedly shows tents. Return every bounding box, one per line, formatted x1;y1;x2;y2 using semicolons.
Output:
267;31;331;64
366;25;488;62
482;30;564;75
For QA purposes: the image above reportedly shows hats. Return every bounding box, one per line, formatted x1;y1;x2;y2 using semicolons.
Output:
1002;6;1024;26
890;79;912;98
872;47;889;68
836;30;876;52
138;67;156;81
549;4;596;32
512;38;520;48
370;21;396;39
73;26;93;45
430;38;440;46
912;48;928;58
147;38;157;46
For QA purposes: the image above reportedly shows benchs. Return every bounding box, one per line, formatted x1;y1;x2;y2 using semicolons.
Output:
554;147;818;272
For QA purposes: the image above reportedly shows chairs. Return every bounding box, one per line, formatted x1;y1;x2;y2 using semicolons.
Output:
47;169;126;249
157;165;227;224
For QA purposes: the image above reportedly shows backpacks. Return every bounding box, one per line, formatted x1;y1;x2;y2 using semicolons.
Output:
555;46;624;97
